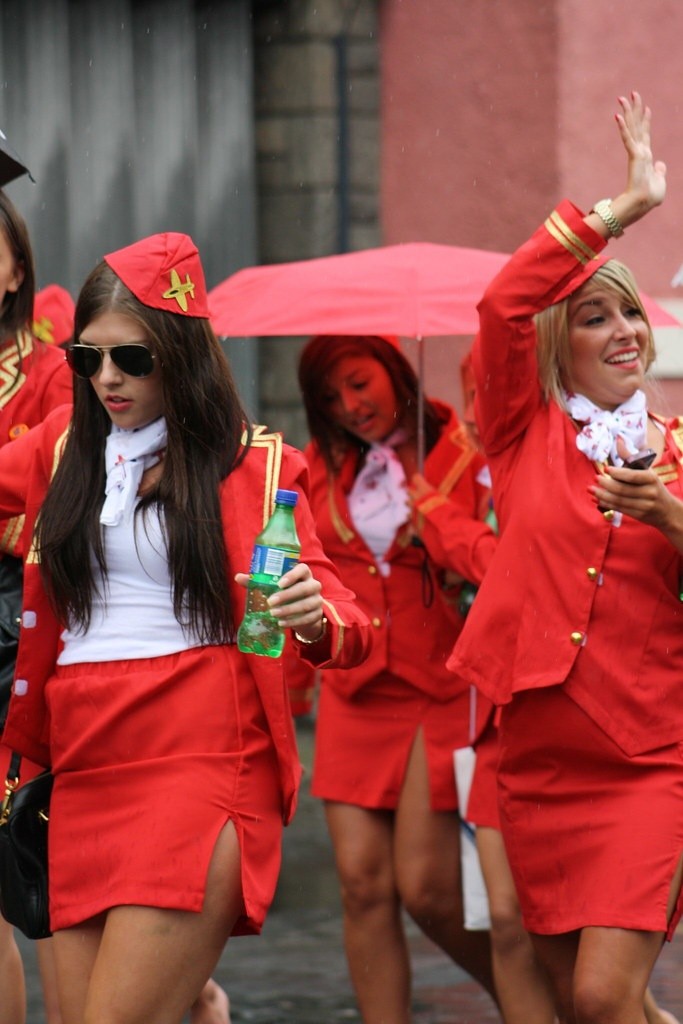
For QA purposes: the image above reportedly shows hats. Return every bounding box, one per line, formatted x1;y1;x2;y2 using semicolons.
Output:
31;284;76;346
555;253;613;301
103;232;210;317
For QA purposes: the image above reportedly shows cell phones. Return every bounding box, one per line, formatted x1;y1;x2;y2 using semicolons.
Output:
597;448;657;513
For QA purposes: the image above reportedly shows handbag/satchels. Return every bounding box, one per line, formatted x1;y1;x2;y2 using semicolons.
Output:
0;769;52;940
452;740;495;933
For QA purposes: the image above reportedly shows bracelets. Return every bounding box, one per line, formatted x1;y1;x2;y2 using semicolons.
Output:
294;615;329;644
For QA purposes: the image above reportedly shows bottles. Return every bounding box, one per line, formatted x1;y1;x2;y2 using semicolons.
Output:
236;488;303;659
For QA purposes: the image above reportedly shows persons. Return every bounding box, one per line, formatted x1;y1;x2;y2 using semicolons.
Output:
0;90;683;1024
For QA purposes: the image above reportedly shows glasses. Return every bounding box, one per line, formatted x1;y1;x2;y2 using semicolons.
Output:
64;343;159;378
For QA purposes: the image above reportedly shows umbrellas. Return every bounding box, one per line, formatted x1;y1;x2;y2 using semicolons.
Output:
206;240;512;610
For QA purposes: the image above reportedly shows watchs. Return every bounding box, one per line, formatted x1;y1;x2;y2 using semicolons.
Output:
589;196;628;239
